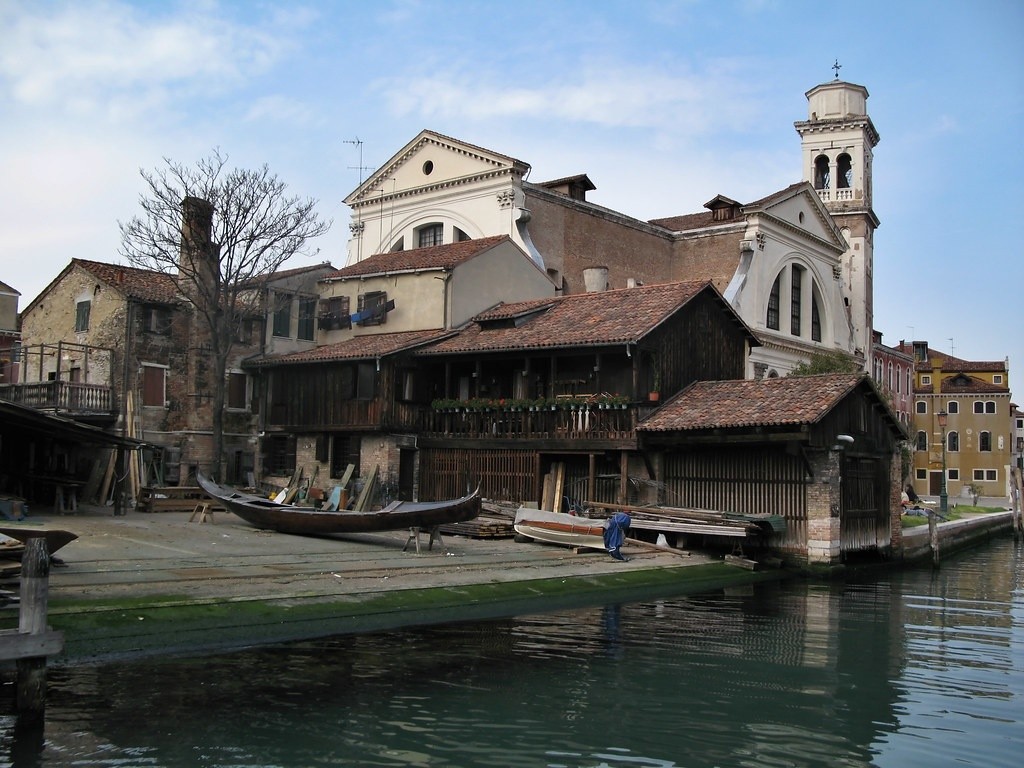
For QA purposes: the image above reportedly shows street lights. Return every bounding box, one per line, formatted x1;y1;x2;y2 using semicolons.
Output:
936;409;949;509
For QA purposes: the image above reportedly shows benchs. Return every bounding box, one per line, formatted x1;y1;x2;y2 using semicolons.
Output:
135;484;228;513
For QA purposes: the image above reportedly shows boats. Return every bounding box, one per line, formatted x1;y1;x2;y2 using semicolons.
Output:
513;504;610;552
196;472;484;537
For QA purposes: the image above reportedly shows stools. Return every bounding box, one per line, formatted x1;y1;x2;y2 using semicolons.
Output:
189;501;218;526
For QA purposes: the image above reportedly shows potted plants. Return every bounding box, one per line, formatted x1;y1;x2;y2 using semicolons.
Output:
650;370;660;400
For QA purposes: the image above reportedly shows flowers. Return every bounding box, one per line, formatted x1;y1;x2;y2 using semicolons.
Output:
431;393;629;410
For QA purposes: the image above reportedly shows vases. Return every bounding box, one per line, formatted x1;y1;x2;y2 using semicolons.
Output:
536;406;540;411
466;408;469;412
512;408;515;411
599;404;603;409
474;408;477;412
622;405;627;410
606;403;610;409
552;406;556;409
587;403;592;409
615;405;619;410
580;406;583;409
455;409;459;412
571;404;575;410
486;408;489;412
529;407;533;411
